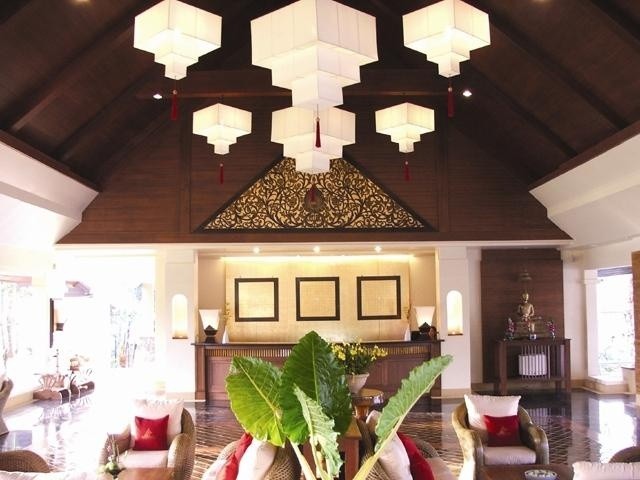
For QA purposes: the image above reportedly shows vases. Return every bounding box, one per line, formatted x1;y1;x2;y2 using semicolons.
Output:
222;325;230;344
347;374;369;394
404;323;411;341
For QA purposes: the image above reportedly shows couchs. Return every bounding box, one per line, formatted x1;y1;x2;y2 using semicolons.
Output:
356;410;457;480
0;378;13;436
451;401;549;479
204;437;299;479
101;397;193;480
0;449;86;479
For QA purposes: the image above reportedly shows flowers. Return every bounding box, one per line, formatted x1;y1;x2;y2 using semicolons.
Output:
330;337;389;374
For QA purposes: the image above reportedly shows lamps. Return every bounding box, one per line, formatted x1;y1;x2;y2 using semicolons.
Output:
444;290;464;336
134;2;221;120
250;0;379;147
376;103;435;183
197;309;222;342
403;1;491;117
170;294;189;340
415;306;435;332
272;109;356;201
193;104;252;183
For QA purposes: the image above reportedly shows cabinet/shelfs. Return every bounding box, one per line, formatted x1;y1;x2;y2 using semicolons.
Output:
493;336;574;396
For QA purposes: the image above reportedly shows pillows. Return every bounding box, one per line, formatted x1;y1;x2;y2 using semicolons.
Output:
134;415;168;450
217;455;239;480
237;438;277;480
573;462;640;480
373;426;410;479
485;415;520;446
399;433;433;480
463;395;522;444
129;397;184;446
235;435;252;465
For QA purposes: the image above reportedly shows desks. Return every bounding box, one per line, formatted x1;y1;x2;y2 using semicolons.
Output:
350;390;385;418
